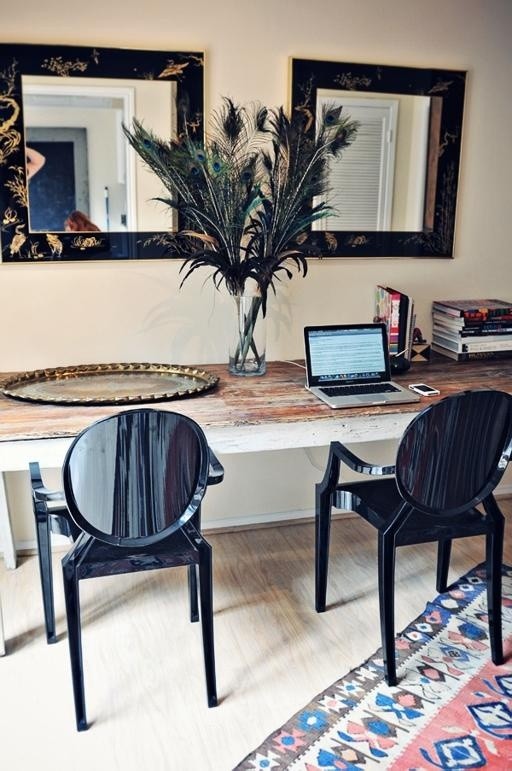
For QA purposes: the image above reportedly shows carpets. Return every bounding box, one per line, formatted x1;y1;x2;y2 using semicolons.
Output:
233;557;511;771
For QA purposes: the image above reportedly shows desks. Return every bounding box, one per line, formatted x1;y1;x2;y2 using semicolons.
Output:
2;358;512;571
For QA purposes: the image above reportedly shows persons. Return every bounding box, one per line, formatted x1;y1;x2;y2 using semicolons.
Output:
64;210;101;233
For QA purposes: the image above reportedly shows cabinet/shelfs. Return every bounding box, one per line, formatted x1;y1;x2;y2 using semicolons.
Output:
313;95;400;232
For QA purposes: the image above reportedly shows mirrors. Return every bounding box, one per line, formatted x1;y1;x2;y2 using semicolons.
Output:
288;54;468;263
1;42;205;262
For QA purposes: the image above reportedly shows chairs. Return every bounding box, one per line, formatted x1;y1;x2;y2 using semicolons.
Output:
314;388;512;686
29;407;226;729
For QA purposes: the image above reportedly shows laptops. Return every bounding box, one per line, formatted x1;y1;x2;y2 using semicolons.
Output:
302;322;421;410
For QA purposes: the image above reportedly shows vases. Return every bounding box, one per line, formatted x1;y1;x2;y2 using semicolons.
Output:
227;296;268;378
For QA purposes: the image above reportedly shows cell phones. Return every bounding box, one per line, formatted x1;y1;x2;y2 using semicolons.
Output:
409;383;440;398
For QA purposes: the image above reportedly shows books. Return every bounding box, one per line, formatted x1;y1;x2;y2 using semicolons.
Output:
375;285;417;363
431;298;512;362
